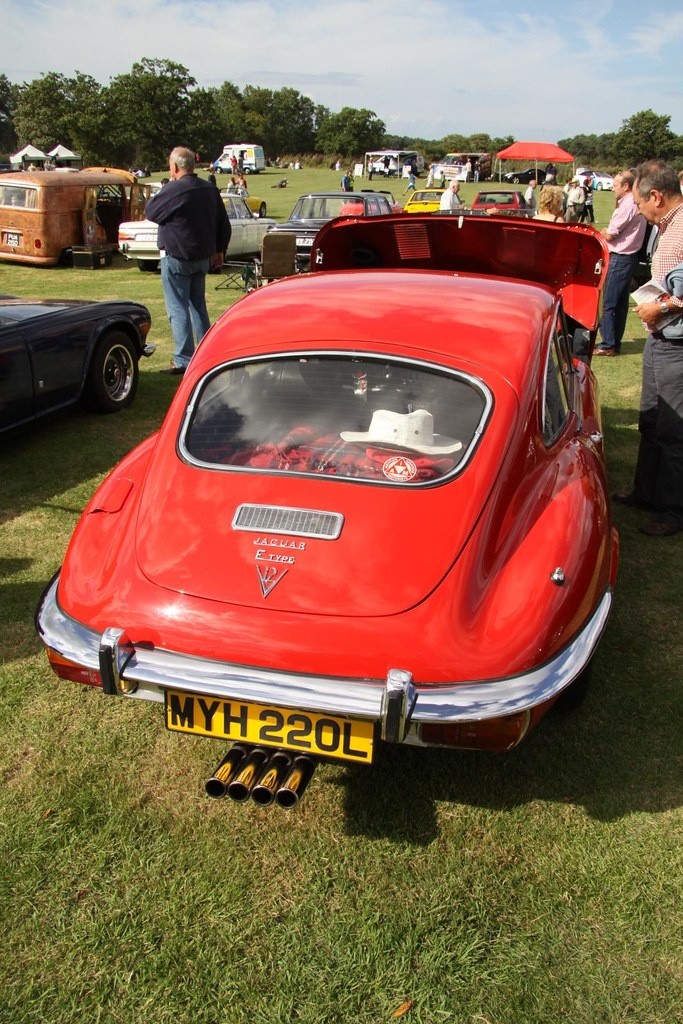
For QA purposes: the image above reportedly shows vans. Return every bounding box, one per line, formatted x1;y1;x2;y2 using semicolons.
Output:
0;167;153;268
438;153;491;181
211;143;266;175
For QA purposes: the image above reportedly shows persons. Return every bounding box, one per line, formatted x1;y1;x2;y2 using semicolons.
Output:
368;157;374;180
278;178;288;188
593;167;683;356
401;171;418;196
424;165;434;188
129;167;151;177
267;157;303;170
340;170;354;204
611;162;683;536
474;159;480;183
207;169;216;185
329;160;343;170
145;147;231;374
439;171;446;187
226;172;247;194
524;179;596;223
440;180;464;210
231;154;243;174
196;152;201;167
28;163;36;172
465;158;472;182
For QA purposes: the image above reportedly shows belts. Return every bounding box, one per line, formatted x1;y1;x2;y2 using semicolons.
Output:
568;205;574;207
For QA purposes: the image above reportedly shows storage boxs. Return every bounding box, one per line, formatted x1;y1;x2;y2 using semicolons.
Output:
73;248;111;269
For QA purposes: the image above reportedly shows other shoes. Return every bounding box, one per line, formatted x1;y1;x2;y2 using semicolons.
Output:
640;521;680;537
160;366;186;375
613;492;635;504
593;343;621;356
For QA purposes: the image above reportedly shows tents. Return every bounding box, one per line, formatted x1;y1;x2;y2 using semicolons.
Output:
494;142;575;185
9;143;85;169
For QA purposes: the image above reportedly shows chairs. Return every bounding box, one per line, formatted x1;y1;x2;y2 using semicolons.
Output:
254;232;298;289
214;252;255;291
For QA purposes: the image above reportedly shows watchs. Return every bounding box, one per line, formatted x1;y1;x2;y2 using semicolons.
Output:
661;302;669;315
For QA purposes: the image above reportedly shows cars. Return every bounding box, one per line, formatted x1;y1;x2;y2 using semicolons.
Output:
503;167;548;184
0;289;159;457
402;188;467;215
267;191;393;263
218;188;268;218
116;193;280;271
126;182;163;201
571;171;614;192
336;188;407;220
33;209;621;811
470;189;528;220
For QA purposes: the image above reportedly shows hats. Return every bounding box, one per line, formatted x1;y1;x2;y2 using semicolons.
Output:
340;409;462;455
569;177;579;184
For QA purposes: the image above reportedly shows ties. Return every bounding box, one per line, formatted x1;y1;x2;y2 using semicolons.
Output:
456;193;461;204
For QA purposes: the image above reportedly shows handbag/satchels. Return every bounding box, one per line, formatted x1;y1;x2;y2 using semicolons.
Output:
573;202;584;212
